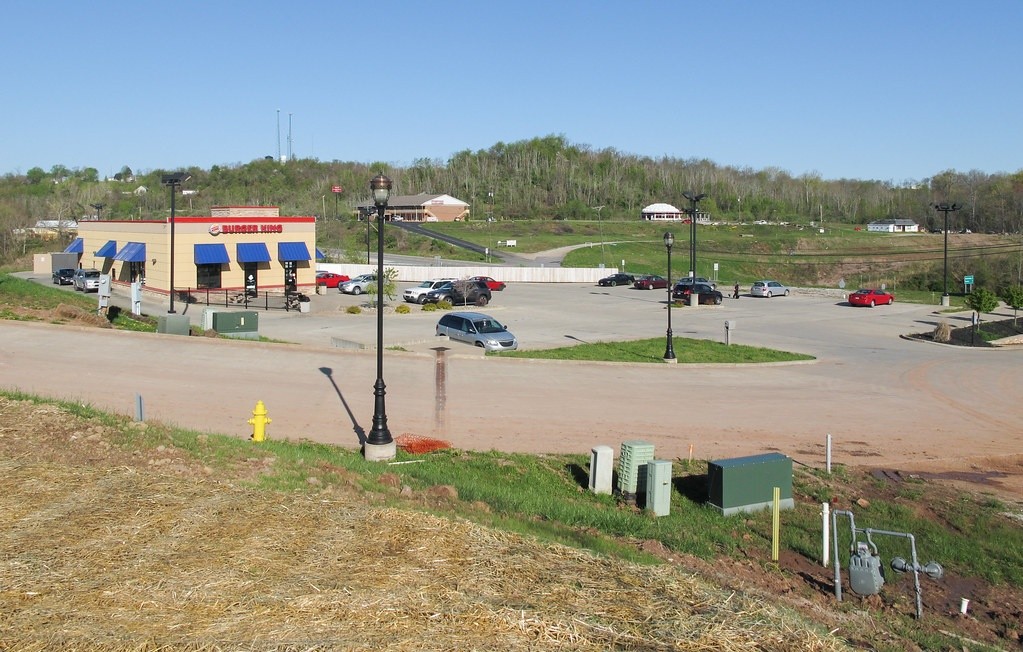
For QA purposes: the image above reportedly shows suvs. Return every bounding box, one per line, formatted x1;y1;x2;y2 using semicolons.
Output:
403;278;491;308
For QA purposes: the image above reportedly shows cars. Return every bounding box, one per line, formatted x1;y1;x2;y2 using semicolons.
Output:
339;274;389;295
672;277;723;305
52;268;76;286
849;289;894;308
469;276;506;291
634;275;668;290
750;281;789;298
758;220;767;224
599;273;635;287
316;271;350;288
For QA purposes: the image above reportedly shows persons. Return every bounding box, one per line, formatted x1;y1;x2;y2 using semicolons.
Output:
733;282;739;298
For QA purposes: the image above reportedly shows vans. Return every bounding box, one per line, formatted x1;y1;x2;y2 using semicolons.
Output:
435;312;519;353
72;268;99;294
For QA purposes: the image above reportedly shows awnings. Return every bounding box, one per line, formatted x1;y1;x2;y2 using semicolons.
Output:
237;243;272;263
194;244;230;264
64;239;82;254
94;241;116;258
112;242;146;262
278;241;311;261
316;248;325;259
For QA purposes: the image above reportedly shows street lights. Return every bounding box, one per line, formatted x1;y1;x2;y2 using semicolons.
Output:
663;231;677;363
683;192;707;276
161;173;192;313
935;202;963;305
94;203;103;220
365;168;397;461
357;205;376;264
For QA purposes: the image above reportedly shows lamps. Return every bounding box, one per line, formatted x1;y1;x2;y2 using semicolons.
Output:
152;259;156;262
93;251;96;253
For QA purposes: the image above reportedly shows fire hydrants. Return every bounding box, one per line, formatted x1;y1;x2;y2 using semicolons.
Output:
248;401;272;441
